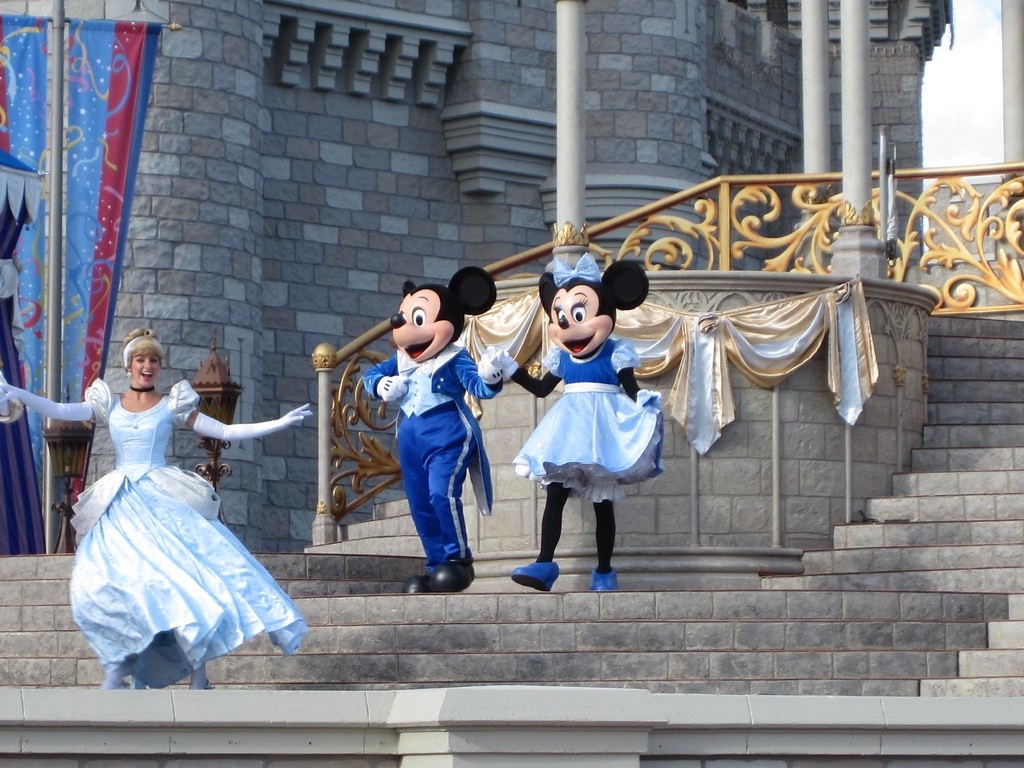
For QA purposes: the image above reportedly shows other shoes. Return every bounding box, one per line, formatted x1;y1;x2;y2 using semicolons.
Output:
191;678;211;691
102;677;131;691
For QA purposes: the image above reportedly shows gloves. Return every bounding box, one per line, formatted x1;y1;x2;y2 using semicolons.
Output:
193;404;314;438
0;373;93;422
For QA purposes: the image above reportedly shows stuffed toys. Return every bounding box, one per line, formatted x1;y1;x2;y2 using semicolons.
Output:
363;266;504;595
496;252;664;593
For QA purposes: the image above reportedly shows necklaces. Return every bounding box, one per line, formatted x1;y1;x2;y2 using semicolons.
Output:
130;385;154;392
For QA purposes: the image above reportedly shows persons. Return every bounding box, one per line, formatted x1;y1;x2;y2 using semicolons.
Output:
0;328;313;690
